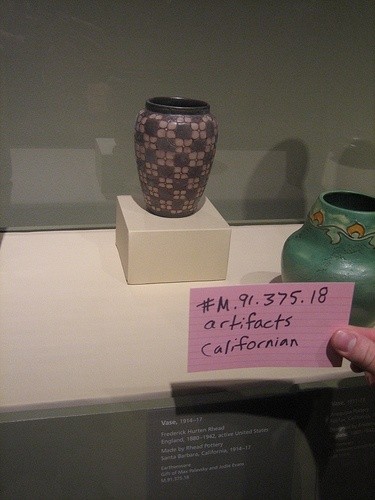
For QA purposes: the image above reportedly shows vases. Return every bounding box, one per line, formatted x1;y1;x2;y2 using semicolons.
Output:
281;189;375;330
133;94;220;220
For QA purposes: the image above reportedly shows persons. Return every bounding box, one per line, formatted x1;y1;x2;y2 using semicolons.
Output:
329;325;374;390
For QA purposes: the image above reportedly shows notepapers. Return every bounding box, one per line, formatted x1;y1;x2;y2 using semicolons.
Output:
190;281;355;375
